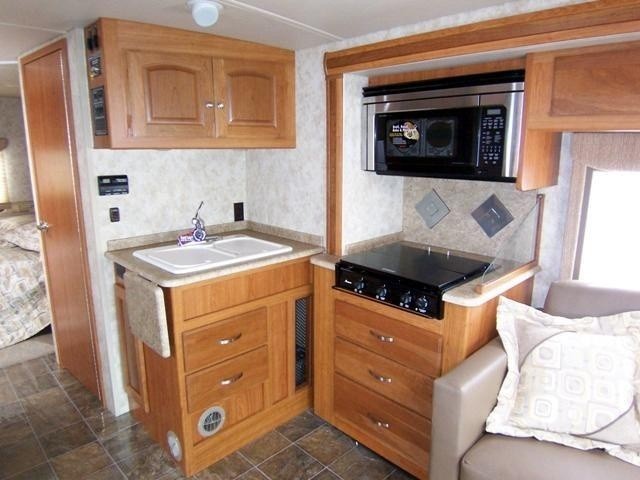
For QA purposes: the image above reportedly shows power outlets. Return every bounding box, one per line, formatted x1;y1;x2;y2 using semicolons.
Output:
110;208;121;222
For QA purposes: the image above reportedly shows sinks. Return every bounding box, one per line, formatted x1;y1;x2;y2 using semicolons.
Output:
210;232;294;262
132;244;240;277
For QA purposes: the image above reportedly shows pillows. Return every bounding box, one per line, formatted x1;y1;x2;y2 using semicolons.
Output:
1;220;41;252
486;296;640;467
0;213;34;234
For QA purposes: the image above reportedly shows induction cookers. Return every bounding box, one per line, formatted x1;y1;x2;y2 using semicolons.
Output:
336;241;489;290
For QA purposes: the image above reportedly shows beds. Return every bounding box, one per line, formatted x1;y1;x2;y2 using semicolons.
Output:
0;206;52;348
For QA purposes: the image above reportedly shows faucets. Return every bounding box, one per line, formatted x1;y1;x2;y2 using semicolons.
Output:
176;200;223;246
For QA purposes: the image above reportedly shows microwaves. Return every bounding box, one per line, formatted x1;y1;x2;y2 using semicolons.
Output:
362;81;525;185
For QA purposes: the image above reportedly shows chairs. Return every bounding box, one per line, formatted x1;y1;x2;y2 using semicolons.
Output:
426;282;640;480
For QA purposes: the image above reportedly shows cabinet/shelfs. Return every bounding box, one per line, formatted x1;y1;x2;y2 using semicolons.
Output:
100;17;297;148
113;257;313;478
314;269;534;480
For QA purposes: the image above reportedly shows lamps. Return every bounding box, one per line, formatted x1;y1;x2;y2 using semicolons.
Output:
185;1;224;28
